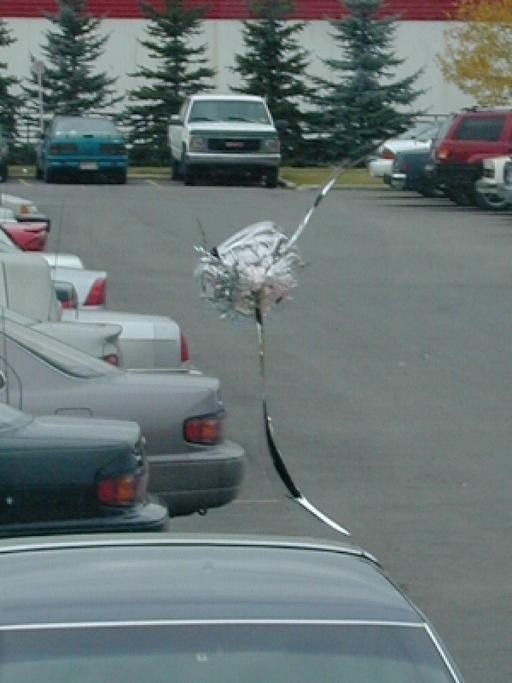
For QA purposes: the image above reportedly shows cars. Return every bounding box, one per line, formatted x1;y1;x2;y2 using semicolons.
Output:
0;399;170;539
0;531;468;683
34;114;128;182
0;317;248;519
0;192;209;375
361;104;511;211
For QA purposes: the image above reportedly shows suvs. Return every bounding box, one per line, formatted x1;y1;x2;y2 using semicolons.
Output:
165;94;283;186
